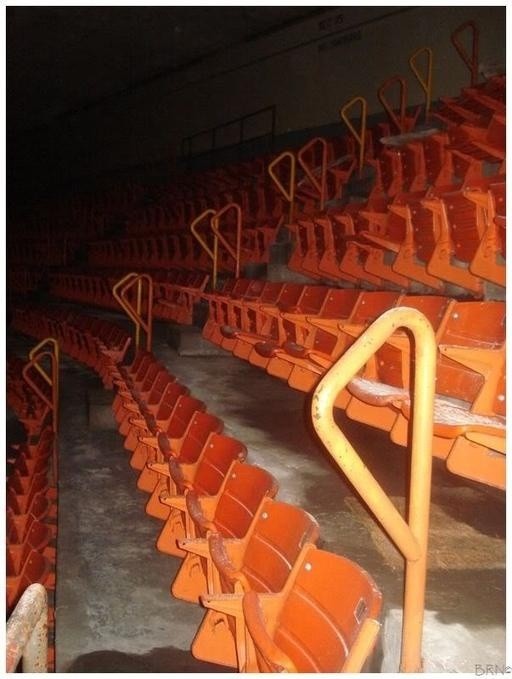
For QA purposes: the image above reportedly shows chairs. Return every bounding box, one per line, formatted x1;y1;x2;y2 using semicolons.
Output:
6;72;505;674
